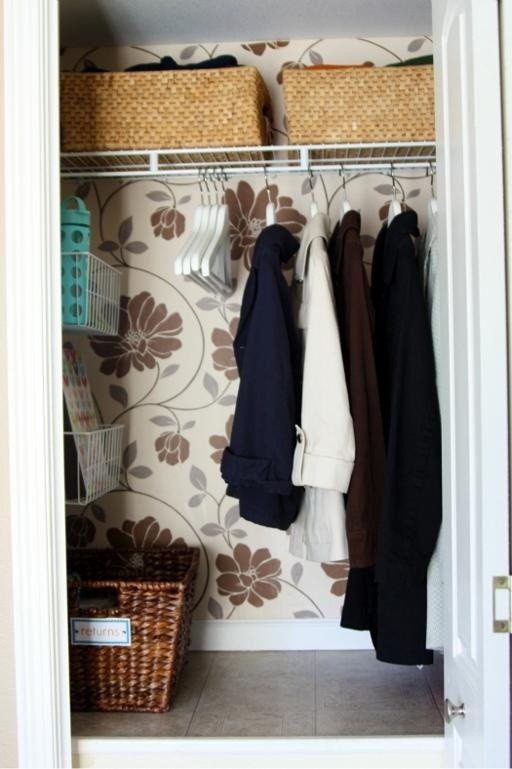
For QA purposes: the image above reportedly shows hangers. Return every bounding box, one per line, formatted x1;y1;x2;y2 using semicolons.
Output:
264;160;439;247
174;166;232;295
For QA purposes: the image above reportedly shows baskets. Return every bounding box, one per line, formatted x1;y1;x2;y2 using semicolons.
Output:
65;424;124;507
282;64;436;166
61;65;272;171
62;251;122;336
68;546;200;714
